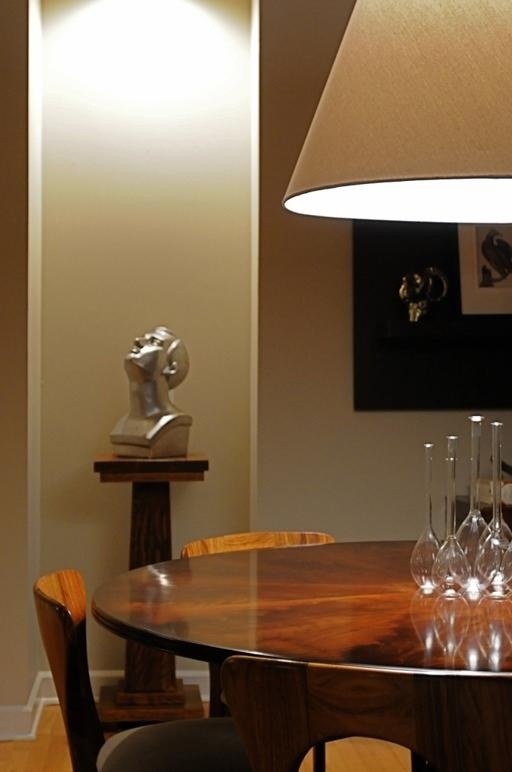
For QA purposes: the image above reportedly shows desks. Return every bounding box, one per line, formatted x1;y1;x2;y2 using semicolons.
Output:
83;539;512;772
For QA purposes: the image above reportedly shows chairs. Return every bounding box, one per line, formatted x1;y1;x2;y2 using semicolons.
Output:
27;566;243;772
182;529;335;772
220;653;512;772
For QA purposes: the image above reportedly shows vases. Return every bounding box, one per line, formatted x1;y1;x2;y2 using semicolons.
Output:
408;414;512;597
408;589;512;671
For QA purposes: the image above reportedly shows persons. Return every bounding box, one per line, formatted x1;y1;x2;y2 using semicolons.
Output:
110;325;195;458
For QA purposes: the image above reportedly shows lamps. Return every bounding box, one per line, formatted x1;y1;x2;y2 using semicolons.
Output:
278;0;512;229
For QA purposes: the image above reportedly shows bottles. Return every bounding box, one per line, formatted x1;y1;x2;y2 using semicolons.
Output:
409;588;511;672
410;416;511;597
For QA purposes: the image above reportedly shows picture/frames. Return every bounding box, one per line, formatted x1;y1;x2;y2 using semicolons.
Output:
445;222;512;319
